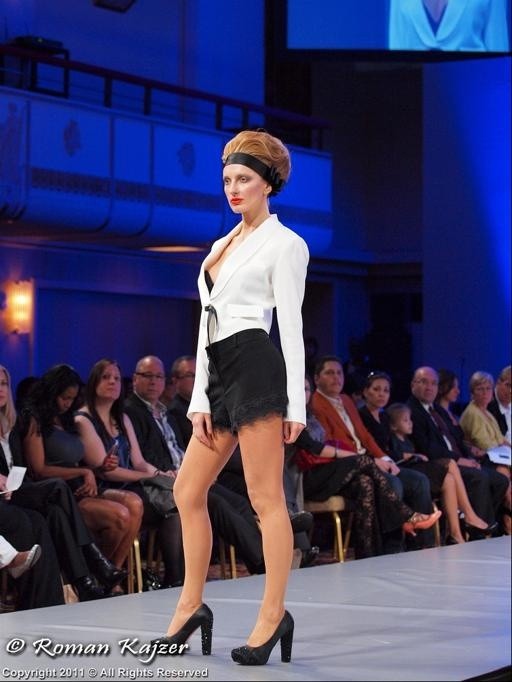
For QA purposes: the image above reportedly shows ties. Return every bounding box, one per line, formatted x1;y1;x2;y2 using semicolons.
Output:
428;405;460;452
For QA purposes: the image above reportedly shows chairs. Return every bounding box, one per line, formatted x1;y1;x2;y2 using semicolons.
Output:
125;497;447;594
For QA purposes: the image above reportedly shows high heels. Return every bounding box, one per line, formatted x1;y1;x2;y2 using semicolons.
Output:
464;522;499;540
151;603;212;655
445;534;458;545
231;609;294;665
402;510;442;537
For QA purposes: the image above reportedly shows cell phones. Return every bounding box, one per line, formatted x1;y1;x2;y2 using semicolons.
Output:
109;438;120;455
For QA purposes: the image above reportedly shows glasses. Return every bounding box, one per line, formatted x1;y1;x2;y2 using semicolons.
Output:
136;373;167;381
414;379;439;387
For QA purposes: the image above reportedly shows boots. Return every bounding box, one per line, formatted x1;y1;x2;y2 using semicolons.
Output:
82;543;131;590
76;576;122;601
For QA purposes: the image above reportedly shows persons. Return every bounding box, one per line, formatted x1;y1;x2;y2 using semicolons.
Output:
0;355;512;614
150;130;310;665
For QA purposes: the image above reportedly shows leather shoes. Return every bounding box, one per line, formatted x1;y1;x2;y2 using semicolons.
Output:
288;508;314;534
7;544;41;579
